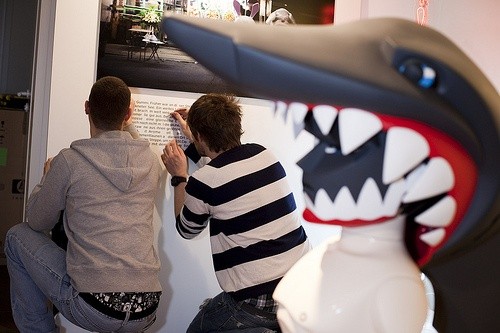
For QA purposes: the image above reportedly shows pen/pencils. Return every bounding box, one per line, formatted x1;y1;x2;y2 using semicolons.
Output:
170;108;187;114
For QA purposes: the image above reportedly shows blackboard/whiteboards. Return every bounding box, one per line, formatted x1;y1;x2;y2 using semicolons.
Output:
23;0;362;333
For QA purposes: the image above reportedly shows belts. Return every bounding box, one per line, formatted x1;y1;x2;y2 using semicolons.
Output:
78;292;158;321
252;308;277;321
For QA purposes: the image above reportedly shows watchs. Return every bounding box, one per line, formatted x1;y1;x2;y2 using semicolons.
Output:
171;176;186;186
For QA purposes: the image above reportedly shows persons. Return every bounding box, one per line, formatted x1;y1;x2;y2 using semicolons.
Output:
3;76;163;333
160;95;313;333
